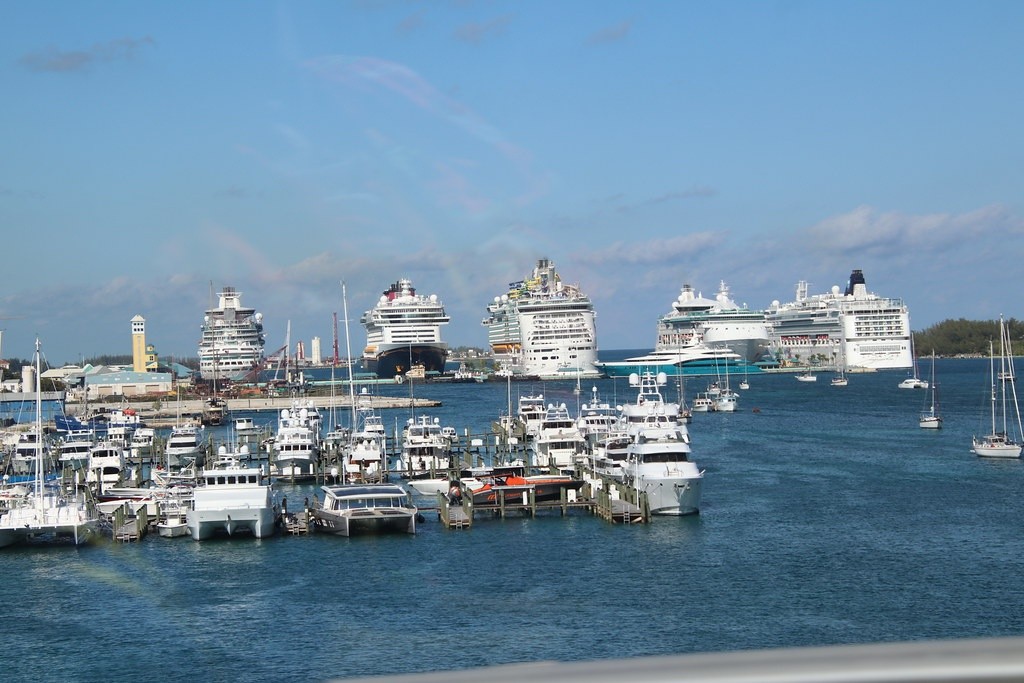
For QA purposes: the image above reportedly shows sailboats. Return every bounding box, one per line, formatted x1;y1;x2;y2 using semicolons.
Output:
918;347;943;430
971;312;1023;458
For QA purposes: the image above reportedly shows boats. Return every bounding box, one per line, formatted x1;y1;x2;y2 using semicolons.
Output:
739;356;749;390
829;347;848;386
480;258;604;381
196;285;265;381
358;277;452;378
762;269;915;374
794;349;817;383
1;279;707;545
691;341;741;414
898;331;929;389
653;278;770;367
